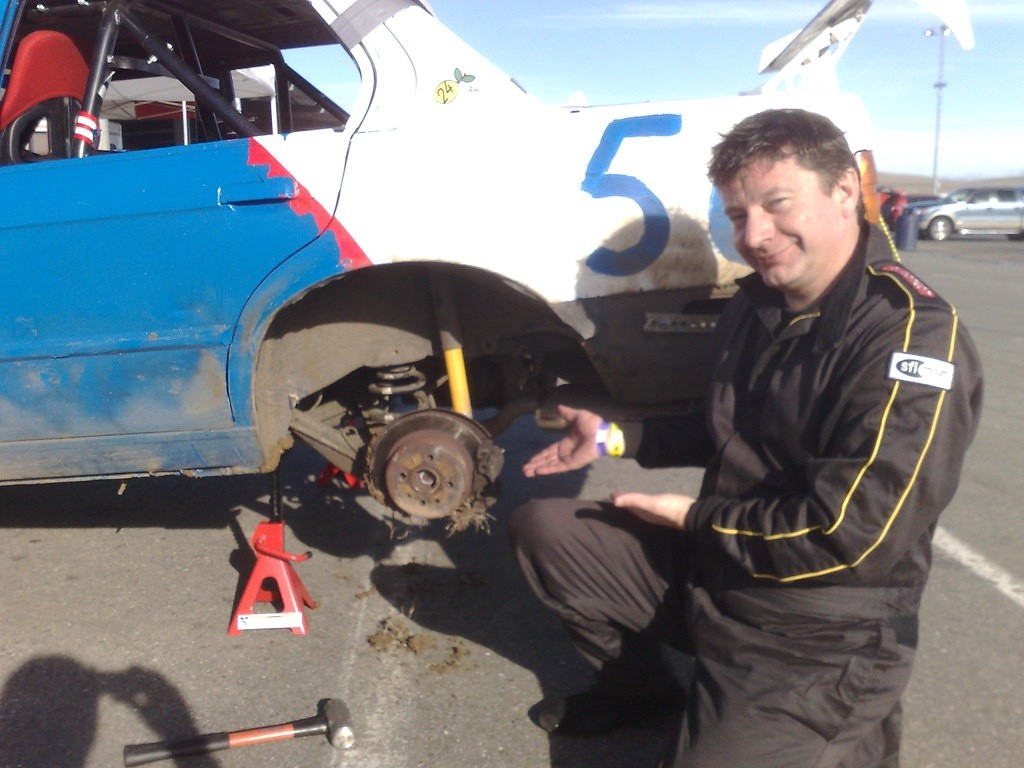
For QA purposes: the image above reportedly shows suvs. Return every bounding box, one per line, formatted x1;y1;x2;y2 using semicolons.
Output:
878;187;1023;243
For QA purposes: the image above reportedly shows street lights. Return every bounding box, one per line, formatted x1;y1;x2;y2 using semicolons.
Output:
925;24;950;196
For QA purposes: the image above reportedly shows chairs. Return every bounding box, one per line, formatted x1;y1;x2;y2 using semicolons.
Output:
0;31;101;165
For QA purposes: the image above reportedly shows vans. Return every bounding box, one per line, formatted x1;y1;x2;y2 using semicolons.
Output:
1;0;875;533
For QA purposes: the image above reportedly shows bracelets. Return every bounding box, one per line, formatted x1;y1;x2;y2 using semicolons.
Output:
595;420;625;457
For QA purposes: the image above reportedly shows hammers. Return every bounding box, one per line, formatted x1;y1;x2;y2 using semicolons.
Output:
122;698;355;768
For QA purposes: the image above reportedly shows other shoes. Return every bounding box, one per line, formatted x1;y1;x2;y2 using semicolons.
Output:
528;688;669;737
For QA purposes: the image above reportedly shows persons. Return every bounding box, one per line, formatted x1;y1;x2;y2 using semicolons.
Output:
506;110;986;768
